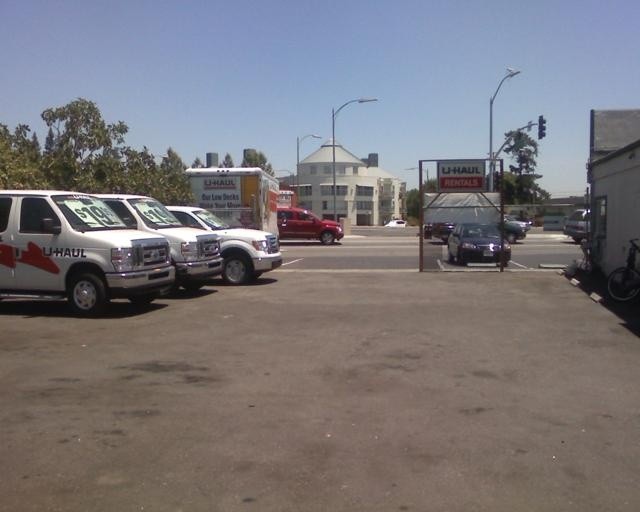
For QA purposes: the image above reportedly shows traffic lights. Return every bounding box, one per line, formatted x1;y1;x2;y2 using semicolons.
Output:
537;116;546;139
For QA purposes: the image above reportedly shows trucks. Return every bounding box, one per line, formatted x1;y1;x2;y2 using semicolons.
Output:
182;168;279;242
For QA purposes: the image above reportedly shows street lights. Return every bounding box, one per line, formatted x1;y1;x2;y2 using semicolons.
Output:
488;69;520;191
296;99;377;220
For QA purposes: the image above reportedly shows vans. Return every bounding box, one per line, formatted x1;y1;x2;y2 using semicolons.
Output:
0;191;284;314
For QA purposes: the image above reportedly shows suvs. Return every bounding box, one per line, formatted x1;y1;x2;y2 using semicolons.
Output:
563;209;592;241
277;207;343;245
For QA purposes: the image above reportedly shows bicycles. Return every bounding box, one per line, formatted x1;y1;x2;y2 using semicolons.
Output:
607;237;639;303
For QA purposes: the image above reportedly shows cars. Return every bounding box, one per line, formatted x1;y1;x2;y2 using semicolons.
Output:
420;214;532;267
385;219;406;228
